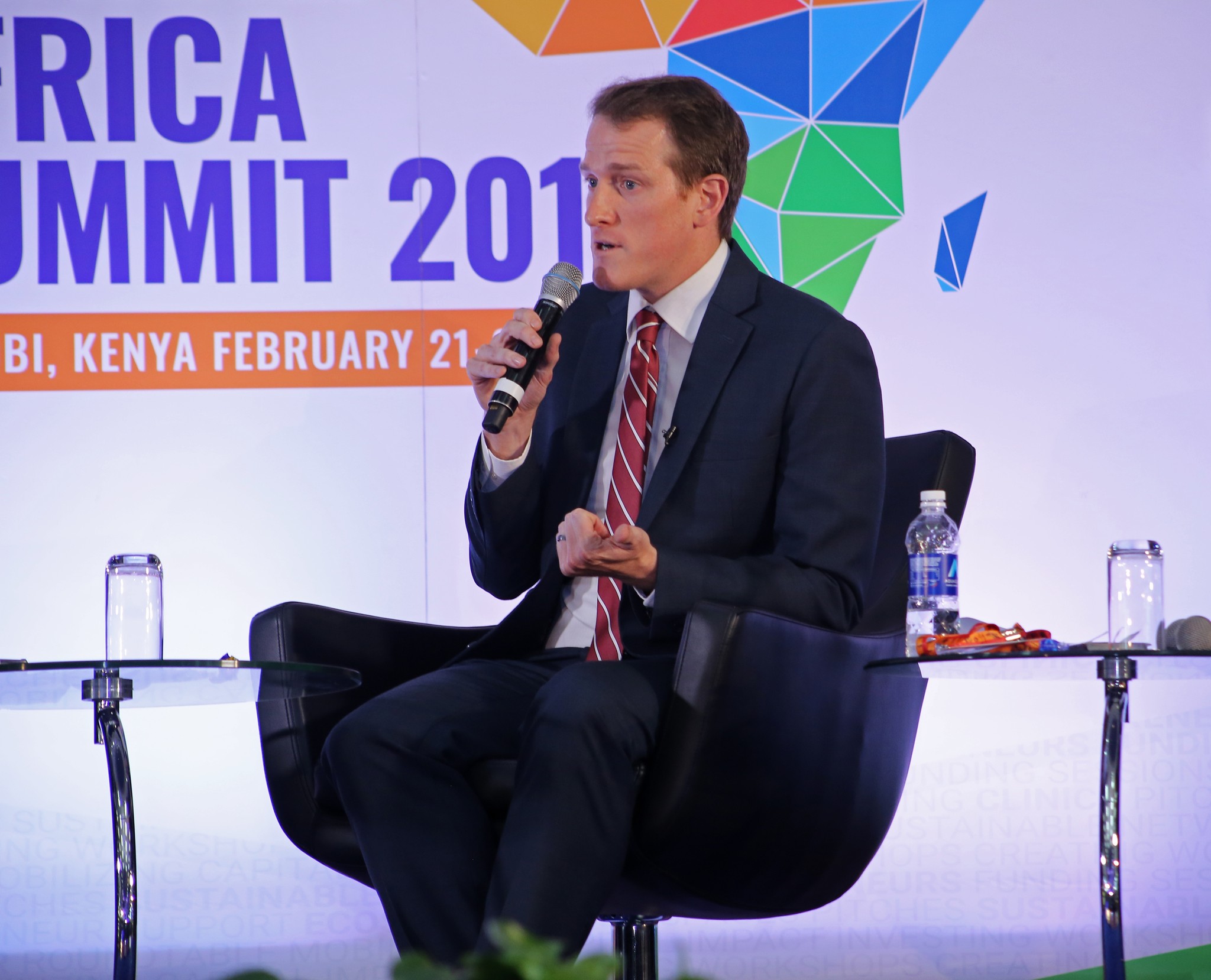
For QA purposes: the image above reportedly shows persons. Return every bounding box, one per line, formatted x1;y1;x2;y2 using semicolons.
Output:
327;78;884;980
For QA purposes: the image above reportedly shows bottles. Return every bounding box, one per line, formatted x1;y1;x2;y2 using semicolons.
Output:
1108;539;1169;652
906;489;964;658
105;553;164;660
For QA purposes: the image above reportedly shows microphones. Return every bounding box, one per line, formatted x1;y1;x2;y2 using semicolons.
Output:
481;262;583;434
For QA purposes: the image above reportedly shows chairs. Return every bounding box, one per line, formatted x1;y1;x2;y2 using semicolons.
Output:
249;428;978;980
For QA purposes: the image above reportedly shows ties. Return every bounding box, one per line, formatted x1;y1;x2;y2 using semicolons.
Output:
585;309;666;663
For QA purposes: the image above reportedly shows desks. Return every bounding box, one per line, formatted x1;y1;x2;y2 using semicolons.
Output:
862;642;1211;980
0;659;364;980
